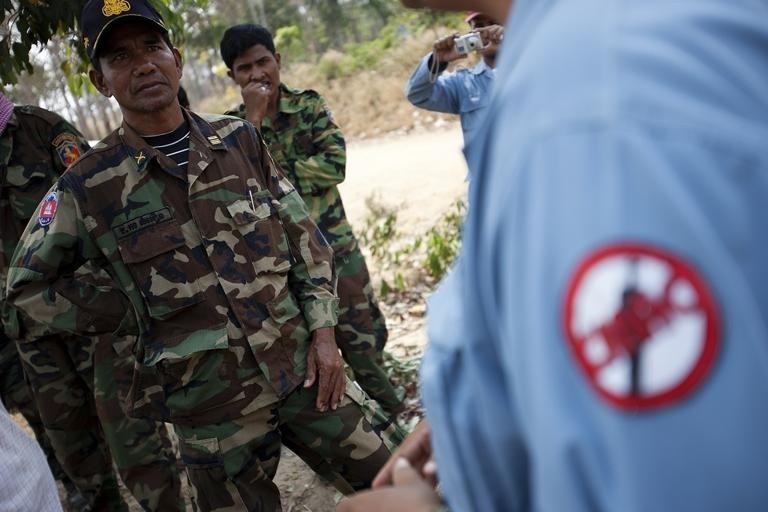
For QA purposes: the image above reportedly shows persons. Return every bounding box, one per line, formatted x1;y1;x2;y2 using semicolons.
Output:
404;9;503;149
0;1;397;512
220;21;406;418
330;0;766;511
0;93;128;512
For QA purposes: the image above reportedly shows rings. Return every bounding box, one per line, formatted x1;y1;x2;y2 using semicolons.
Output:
261;87;266;91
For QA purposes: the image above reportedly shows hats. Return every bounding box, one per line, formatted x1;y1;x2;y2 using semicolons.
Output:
78;1;170;61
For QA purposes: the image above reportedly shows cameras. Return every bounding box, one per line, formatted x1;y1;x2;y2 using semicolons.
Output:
452;32;484;56
